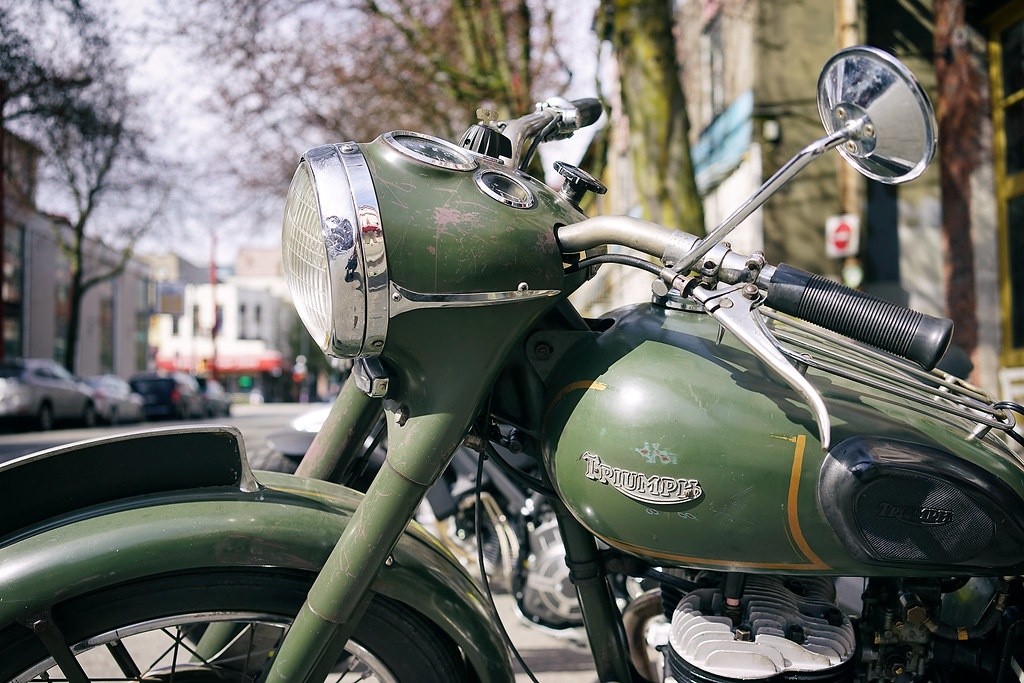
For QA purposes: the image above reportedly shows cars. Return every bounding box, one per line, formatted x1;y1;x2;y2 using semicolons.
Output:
81;371;147;426
0;358;99;433
193;376;232;420
126;370;210;422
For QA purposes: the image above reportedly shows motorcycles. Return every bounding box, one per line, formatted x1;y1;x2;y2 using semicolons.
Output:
0;51;1024;683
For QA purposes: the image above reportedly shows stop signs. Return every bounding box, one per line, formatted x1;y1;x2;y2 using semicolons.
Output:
831;221;852;251
